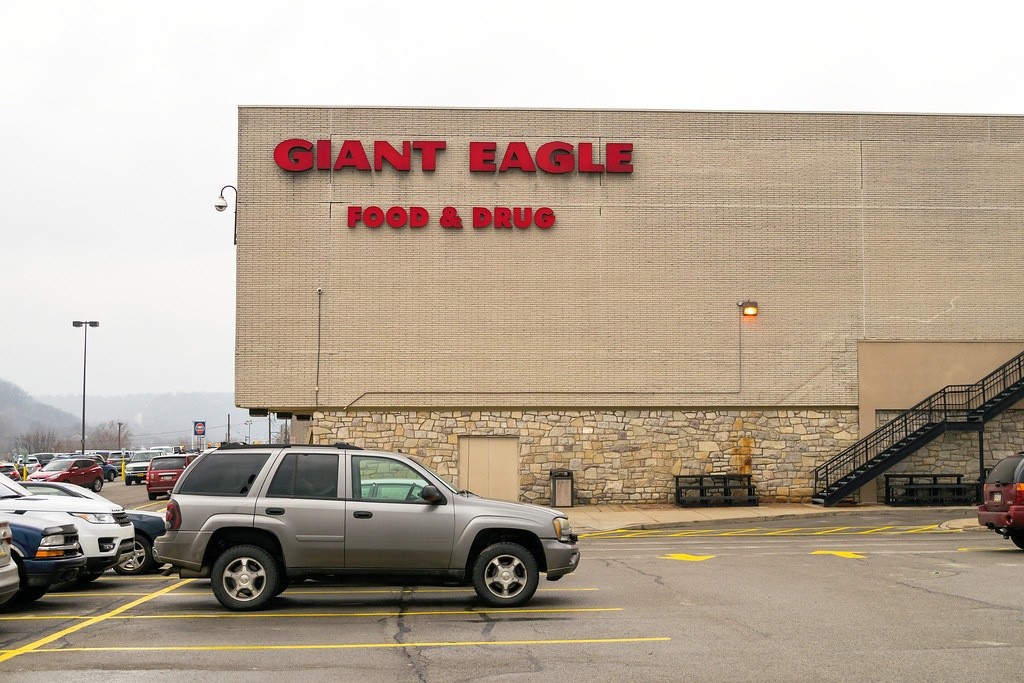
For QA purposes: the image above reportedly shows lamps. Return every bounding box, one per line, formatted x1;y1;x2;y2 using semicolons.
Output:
741;299;758;316
215;185;239;244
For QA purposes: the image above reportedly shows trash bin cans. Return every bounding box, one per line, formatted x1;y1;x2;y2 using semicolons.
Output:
550;468;574;508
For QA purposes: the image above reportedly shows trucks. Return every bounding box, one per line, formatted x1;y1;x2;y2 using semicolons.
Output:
150;446;180;455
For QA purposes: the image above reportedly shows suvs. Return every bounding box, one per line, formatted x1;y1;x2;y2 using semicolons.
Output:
152;441;581;611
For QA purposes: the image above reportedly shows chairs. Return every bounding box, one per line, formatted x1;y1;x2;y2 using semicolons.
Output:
269;460;295;496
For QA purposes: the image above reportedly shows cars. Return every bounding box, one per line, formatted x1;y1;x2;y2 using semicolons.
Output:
105;452;131;472
57;453;118;482
0;472;136;581
33;453;54;468
26;459;104;493
12;454;41;474
15;481;167;575
359;479;429;501
0;519;21;606
0;465;22;481
124;450;167;486
146;454;199;500
977;451;1024;551
0;513;88;604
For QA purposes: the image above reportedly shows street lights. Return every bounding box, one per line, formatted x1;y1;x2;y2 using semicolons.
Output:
245;420;252;445
73;321;99;455
118;422;123;451
200;437;204;450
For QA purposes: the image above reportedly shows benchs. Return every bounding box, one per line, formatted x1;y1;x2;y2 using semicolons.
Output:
883;474;972;506
673;473;761;509
